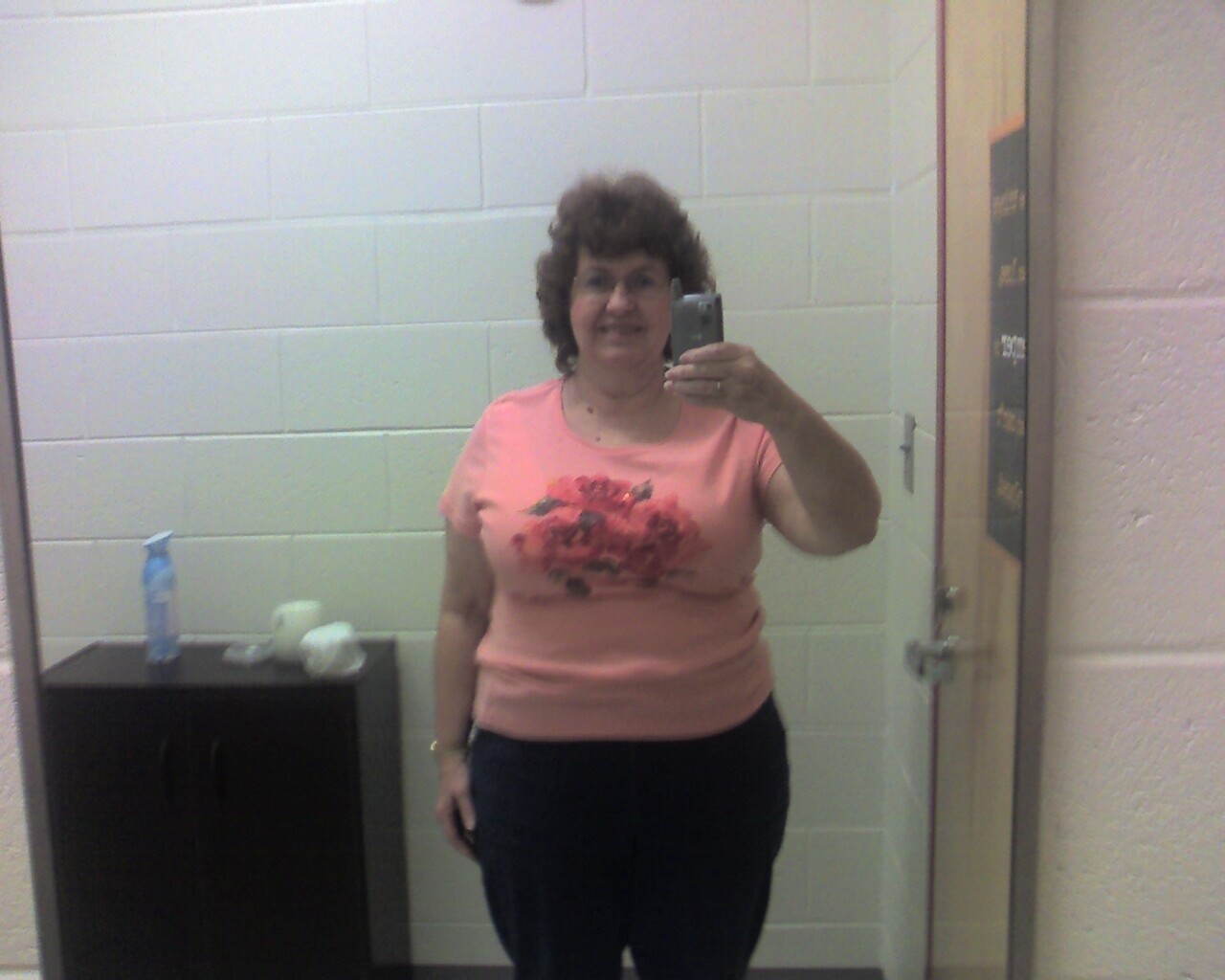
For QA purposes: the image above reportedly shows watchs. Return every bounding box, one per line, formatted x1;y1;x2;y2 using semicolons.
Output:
428;739;470;766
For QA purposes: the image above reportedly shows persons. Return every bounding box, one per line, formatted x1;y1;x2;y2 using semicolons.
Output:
429;165;884;980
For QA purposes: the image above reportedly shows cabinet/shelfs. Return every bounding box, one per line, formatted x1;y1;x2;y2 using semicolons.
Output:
39;634;410;979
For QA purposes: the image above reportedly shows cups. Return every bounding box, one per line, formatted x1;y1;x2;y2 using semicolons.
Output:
270;599;321;666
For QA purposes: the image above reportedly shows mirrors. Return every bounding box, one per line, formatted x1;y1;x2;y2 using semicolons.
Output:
0;1;1224;980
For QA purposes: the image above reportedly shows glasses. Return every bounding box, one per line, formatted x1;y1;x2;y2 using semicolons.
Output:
571;267;672;298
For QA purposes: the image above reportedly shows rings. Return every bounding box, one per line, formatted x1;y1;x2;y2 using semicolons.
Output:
715;379;722;398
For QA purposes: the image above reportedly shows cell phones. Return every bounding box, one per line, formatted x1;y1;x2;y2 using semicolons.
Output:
669;279;725;365
